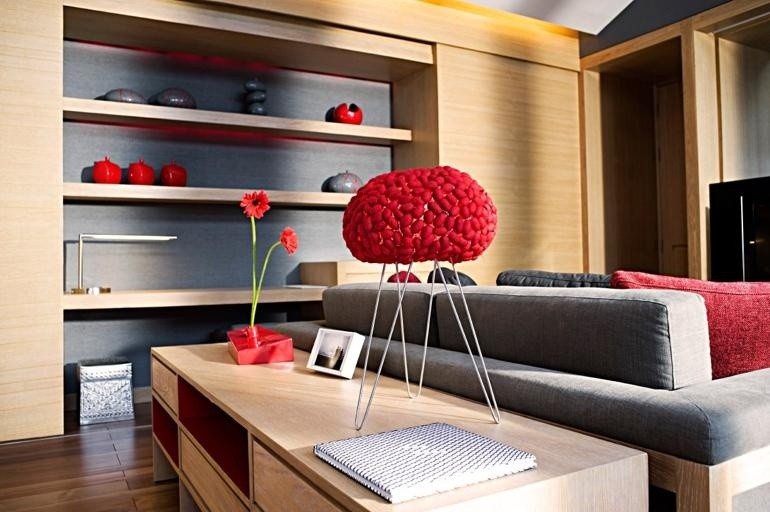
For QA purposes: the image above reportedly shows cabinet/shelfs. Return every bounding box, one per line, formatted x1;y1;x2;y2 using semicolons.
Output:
63;0;440;437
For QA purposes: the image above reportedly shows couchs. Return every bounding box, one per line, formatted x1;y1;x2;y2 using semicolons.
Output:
278;270;769;512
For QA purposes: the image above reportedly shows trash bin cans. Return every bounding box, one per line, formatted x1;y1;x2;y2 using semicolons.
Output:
76;356;135;425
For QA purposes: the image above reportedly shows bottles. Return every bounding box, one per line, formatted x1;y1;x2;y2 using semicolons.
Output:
93;156;187;186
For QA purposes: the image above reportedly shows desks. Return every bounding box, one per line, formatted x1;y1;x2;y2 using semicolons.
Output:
148;343;649;512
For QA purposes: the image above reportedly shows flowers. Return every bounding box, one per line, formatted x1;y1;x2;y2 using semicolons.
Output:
239;188;298;329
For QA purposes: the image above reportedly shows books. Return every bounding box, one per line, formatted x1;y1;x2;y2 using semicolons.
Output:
313;419;540;505
282;284;329;290
